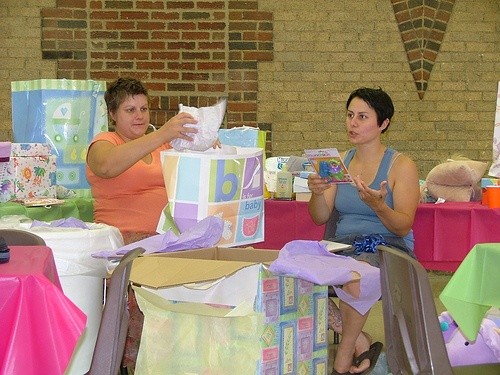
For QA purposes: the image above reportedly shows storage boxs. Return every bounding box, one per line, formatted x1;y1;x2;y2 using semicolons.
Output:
0;162;15;203
0;141;11;162
11;142;53;157
11;155;57;199
107;242;378;375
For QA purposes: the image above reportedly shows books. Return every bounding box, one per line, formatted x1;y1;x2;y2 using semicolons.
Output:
305;148;354;184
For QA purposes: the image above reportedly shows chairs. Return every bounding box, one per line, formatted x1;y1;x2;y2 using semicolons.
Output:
84;246;147;375
375;241;457;375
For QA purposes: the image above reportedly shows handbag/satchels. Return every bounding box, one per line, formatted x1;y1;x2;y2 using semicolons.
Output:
156;143;265;247
217;125;268;199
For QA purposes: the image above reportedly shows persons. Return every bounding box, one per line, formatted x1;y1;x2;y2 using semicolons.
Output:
87;76;222;375
308;86;421;375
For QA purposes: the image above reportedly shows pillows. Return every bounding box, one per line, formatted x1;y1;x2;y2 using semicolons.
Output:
421;155;490;202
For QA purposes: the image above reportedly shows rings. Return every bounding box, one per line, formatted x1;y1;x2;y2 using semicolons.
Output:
359;190;361;192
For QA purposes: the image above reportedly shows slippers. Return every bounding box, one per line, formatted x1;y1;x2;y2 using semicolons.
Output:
332;341;383;375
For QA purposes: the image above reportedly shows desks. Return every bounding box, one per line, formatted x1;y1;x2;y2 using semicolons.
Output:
0;243;89;375
412;199;500;275
0;193;98;222
437;242;500;344
250;196;327;250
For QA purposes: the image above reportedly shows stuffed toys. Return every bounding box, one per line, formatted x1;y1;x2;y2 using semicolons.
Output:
438;310;500;367
